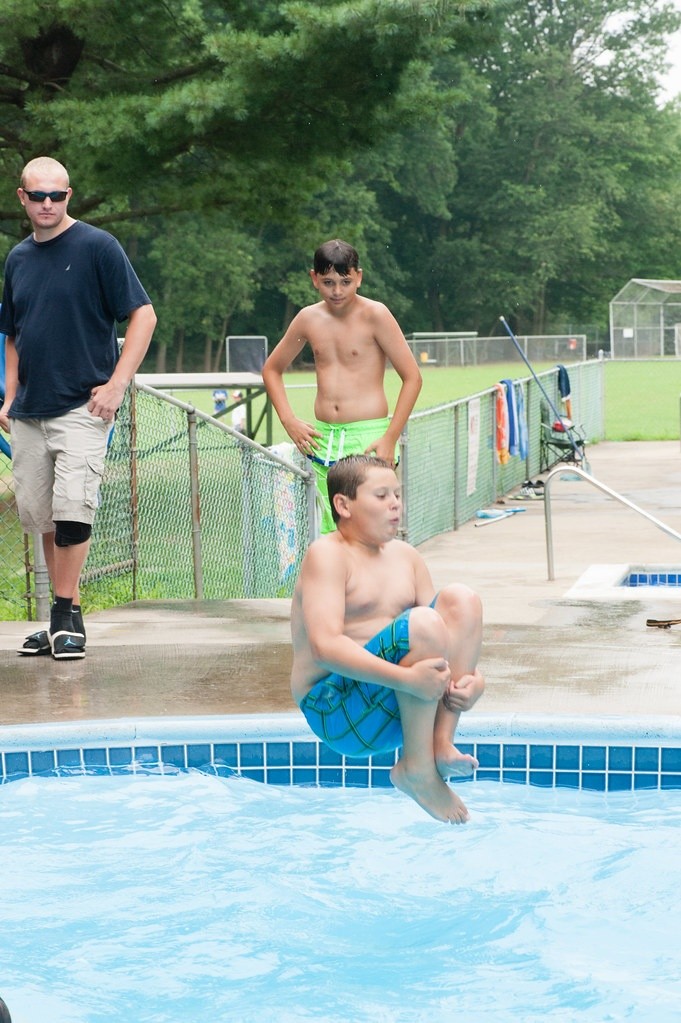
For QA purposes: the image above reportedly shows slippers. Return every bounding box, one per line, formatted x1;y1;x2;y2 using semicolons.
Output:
522;480;539;488
16;631;50;654
47;627;86;658
535;481;544;487
647;620;681;629
508;486;545;501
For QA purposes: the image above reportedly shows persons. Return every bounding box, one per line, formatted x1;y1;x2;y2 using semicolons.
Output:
262;239;423;540
213;388;249;452
290;453;486;825
0;156;158;660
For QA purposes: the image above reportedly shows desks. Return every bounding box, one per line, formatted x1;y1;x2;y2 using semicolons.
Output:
120;371;273;463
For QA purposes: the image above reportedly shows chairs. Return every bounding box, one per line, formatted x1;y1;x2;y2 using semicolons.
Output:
539;404;585;473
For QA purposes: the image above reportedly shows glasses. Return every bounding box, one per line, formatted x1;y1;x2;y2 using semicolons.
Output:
23;189;68;201
